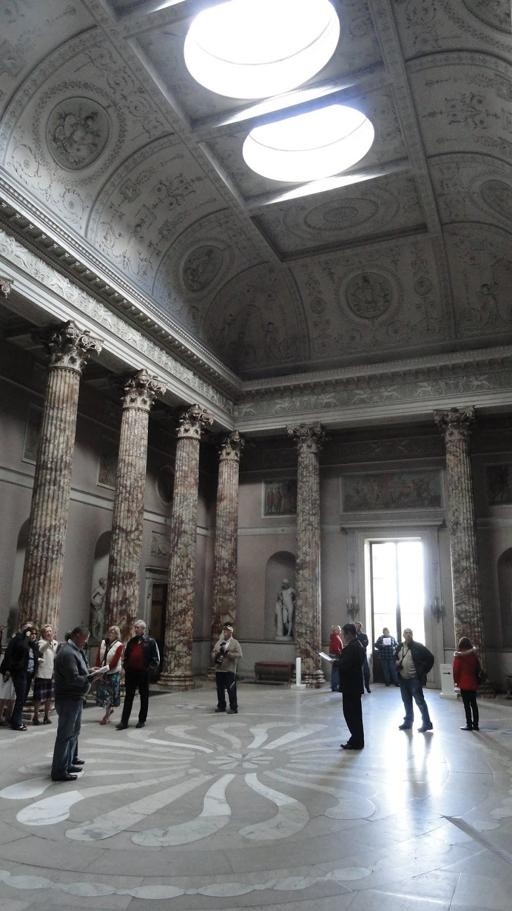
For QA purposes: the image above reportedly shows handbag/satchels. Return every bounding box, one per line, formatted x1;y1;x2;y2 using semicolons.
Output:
476;656;487;686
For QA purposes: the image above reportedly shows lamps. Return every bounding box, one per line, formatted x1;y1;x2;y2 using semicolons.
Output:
346;596;361;622
430;595;445;624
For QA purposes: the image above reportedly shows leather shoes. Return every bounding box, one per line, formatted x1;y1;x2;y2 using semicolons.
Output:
399;722;412;729
461;725;479;731
51;756;85;782
417;724;433;732
340;742;363;750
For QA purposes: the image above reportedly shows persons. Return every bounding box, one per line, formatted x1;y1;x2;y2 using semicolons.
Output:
32;625;57;725
275;578;294;638
115;620;162;730
393;629;435;733
328;623;343;693
353;620;371;694
338;625;365;752
209;621;243;715
95;625;125;726
56;631;87;773
9;626;42;731
48;625;105;782
89;575;108;644
450;636;483;732
373;627;401;688
12;621;34;641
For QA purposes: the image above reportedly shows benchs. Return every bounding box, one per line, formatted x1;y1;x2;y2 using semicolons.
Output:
254;661;295;689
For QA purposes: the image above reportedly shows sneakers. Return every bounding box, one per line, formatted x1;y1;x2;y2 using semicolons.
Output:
10;722;28;732
114;721;128;729
32;716;52;726
136;719;145;728
100;708;115;726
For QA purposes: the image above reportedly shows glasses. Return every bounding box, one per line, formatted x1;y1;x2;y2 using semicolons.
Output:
31;632;38;636
